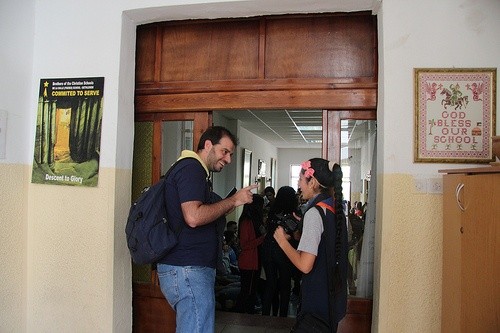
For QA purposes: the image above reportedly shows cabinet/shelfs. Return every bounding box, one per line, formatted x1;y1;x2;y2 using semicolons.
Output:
438;167;500;333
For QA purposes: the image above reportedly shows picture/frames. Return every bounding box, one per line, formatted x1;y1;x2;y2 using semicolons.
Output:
414;67;497;163
242;148;253;188
271;157;276;190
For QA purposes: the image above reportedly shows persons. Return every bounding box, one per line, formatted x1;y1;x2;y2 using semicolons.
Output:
274;158;348;333
215;186;367;318
157;125;260;333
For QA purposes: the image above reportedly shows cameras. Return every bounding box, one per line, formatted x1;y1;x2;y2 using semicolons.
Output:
271;214;298;234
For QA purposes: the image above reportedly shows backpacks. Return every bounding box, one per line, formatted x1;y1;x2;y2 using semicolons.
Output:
125;157;208;265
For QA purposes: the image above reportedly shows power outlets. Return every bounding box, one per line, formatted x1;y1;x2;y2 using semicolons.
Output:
428;177;443;194
